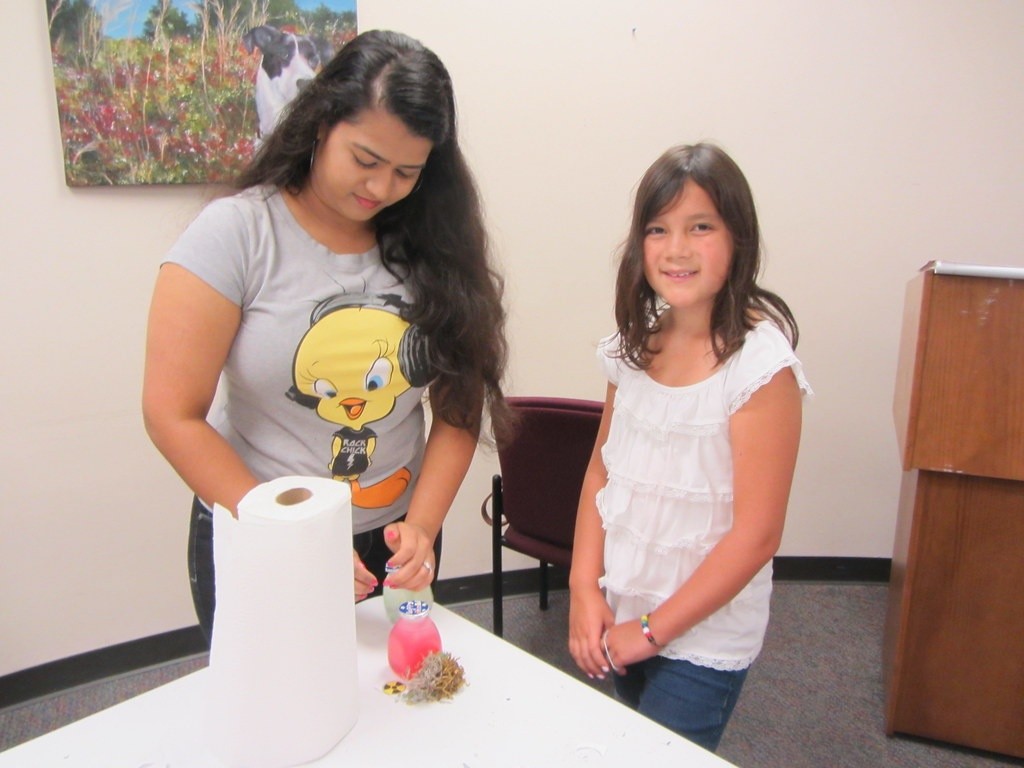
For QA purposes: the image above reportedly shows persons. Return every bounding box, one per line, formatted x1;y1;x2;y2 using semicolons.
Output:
568;142;813;754
143;29;512;644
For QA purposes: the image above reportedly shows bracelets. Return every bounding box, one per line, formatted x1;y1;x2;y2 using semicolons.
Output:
603;629;621;672
641;615;667;649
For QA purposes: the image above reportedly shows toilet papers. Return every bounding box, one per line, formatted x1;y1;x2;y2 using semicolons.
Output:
204;472;362;767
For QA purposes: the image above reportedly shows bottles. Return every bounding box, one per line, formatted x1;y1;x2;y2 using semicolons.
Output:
387;599;442;680
382;562;434;626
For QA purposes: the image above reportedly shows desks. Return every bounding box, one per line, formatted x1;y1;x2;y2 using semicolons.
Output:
1;588;740;768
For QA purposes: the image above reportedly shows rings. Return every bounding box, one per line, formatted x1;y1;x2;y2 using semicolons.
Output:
422;561;432;574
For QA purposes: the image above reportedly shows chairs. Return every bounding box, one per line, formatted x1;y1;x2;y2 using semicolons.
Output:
478;392;605;640
